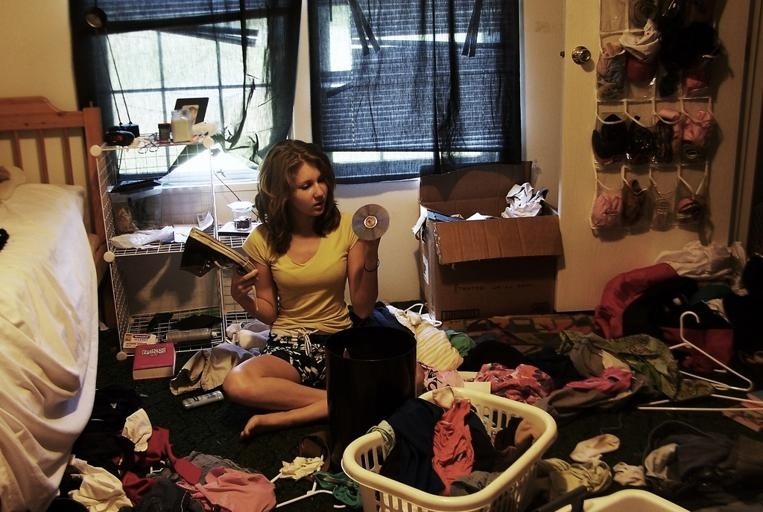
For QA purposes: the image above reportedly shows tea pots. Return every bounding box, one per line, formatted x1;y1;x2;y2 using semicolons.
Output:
226;200;259;232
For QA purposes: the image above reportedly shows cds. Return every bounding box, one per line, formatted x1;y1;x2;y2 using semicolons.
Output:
351;202;390;243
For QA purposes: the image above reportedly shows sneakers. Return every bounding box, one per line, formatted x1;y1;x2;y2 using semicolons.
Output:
179;227;259;283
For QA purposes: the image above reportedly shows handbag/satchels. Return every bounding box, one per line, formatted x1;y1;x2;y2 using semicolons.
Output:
643;419;735;501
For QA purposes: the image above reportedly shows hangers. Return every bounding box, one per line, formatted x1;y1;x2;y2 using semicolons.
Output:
403;302;443;328
637;310;762;413
269;453;347;509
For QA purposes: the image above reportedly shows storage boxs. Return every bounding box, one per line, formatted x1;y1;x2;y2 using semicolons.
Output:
417;160;564;330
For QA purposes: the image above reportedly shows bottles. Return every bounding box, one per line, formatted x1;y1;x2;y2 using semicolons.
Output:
165;327;218;346
158;123;171;145
170;108;194;143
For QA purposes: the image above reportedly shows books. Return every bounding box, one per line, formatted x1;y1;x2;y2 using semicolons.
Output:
130;341;178;382
121;330;156;353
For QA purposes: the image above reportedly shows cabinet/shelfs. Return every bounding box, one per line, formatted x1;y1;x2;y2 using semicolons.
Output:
88;142;271;362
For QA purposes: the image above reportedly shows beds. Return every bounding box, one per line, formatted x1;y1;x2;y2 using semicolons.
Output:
0;95;116;512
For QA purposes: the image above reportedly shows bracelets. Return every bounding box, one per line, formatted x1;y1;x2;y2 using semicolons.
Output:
362;259;381;272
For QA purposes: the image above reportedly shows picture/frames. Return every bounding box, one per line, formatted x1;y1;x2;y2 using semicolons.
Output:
170;97;210;125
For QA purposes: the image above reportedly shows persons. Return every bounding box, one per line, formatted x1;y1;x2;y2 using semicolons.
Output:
221;138;424;438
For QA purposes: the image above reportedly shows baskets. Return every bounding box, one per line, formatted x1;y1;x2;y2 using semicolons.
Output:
341;385;559;511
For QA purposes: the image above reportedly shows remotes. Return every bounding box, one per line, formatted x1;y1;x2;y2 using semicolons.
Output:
183;389;225;409
